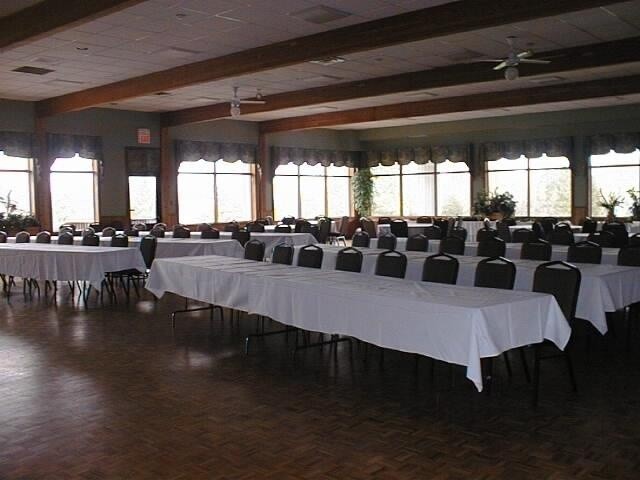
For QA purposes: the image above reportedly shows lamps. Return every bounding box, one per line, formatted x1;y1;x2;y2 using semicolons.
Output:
504;64;520;79
230;103;241;119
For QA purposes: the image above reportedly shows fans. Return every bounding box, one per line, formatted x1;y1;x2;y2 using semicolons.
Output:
466;33;550;80
194;84;267;120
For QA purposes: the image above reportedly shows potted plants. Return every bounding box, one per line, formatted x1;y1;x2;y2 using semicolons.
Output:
476;187;518;221
595;188;626;222
626;187;640;221
